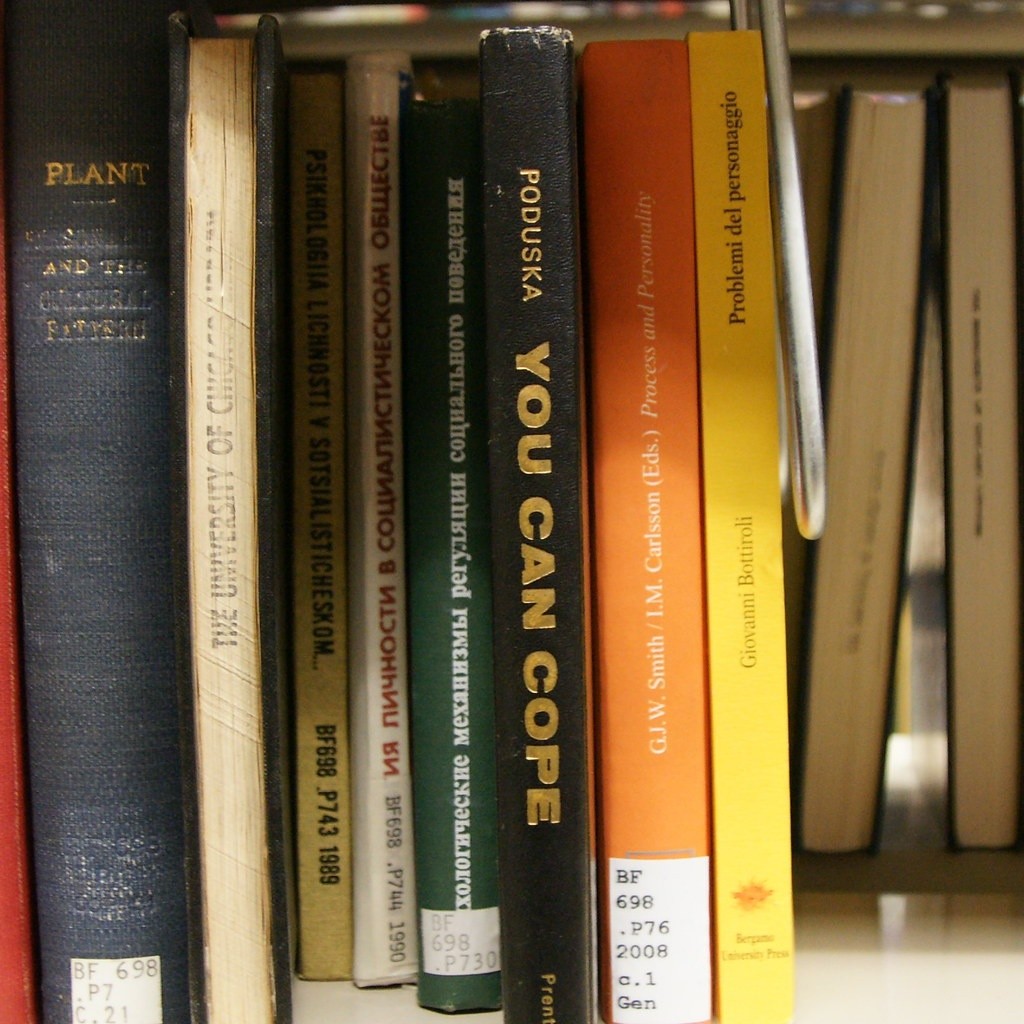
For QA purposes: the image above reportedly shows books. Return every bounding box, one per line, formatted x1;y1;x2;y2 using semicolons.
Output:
1;0;1024;1024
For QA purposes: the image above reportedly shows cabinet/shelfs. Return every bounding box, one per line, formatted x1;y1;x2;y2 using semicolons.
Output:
211;14;1023;1024
